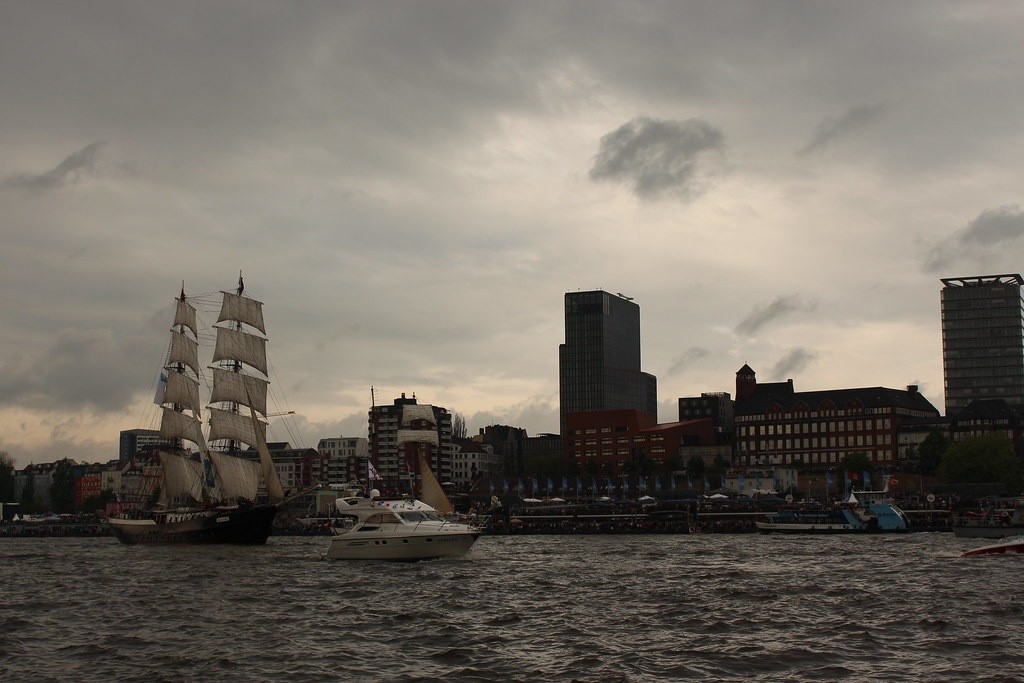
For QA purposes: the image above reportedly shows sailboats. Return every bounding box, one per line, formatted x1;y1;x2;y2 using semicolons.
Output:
99;266;322;547
397;404;485;540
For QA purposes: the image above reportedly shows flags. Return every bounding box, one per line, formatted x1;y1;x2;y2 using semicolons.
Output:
489;465;887;495
369;461;383;480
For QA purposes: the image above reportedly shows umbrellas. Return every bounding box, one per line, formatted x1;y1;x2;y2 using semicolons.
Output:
523;498;565;506
596;495;654;504
704;494;729;499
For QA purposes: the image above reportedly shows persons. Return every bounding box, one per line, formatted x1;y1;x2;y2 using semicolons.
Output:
161;514;207;525
127;505;137;520
765;511;800;524
281;518;332;536
918;499;933;528
384;489;403;497
975;502;1012;526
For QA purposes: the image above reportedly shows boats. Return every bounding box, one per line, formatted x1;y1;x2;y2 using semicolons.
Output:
755;502;911;534
327;489;491;562
949;496;1024;539
